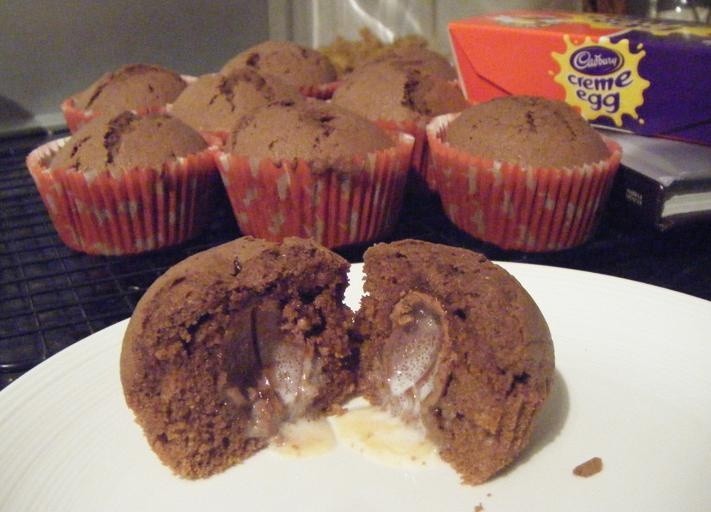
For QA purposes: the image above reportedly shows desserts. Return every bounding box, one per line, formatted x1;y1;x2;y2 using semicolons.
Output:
25;27;623;485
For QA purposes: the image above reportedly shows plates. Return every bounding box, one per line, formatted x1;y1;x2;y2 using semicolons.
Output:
1;260;711;511
